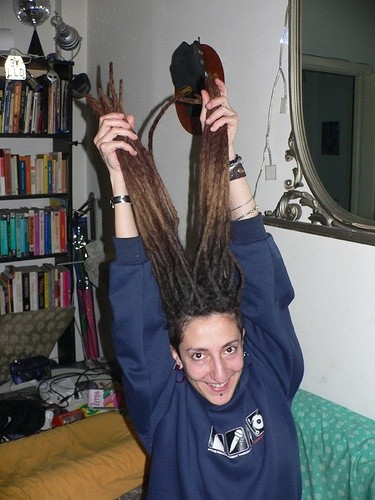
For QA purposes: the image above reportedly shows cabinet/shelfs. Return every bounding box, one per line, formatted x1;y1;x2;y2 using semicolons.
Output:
0;57;76;363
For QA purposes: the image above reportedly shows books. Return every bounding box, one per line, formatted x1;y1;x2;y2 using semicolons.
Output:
0;148;69;196
0;203;68;259
0;263;70;315
0;70;68;134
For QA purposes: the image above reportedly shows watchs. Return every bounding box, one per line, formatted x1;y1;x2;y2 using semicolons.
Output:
109;195;131;211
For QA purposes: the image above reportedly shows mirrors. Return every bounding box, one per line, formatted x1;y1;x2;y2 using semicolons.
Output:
261;0;375;247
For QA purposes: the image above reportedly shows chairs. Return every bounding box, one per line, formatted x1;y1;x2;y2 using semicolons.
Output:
291;389;375;500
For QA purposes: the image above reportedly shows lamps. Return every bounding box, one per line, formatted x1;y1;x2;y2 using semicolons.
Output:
51;15;82;62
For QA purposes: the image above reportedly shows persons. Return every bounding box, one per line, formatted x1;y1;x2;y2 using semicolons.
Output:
81;60;305;499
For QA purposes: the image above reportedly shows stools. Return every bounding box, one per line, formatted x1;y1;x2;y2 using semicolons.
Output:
0;410;149;500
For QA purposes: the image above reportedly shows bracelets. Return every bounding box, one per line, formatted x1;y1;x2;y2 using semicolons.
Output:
229;163;247;181
234;204;258;221
228;196;254;211
229;153;240;166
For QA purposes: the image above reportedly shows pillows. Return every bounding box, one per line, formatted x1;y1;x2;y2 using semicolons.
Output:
0;306;75;385
